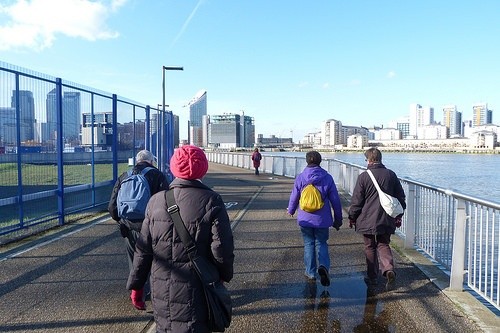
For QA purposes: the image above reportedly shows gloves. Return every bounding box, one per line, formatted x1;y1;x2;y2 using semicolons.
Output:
395;218;402;228
131;289;145;310
332;219;342;231
349;218;355;229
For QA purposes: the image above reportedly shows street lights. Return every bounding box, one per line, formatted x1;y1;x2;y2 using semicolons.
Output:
160;65;184;172
156;103;170;169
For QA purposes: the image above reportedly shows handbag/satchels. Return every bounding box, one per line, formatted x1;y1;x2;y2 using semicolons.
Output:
379;191;403;218
203;280;232;333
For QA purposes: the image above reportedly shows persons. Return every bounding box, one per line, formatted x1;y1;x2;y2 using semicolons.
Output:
107;150;171;299
251;148;262;175
286;152;343;286
348;148;407;290
125;145;234;333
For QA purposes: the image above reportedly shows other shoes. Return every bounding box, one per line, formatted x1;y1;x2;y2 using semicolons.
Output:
318;266;330;287
145;291;152;301
386;270;395;291
304;272;316;284
364;276;378;285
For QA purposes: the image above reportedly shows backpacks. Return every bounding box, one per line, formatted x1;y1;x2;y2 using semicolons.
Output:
116;166;162;220
253;152;260;161
299;184;325;213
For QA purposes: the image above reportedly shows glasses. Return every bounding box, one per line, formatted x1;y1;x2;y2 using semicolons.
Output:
365;158;371;163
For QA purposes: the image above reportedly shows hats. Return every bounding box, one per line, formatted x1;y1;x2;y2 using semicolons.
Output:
170;146;208;180
